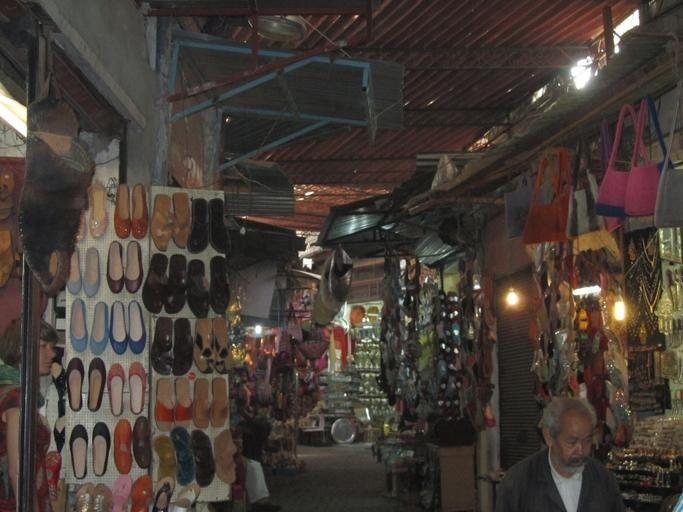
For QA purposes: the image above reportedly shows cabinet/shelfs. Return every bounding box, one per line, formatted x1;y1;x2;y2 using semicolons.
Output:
349;321;393;441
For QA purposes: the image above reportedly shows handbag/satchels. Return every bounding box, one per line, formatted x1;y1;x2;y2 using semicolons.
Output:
503;158;683;244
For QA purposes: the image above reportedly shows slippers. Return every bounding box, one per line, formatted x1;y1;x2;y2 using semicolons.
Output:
143;193;237;511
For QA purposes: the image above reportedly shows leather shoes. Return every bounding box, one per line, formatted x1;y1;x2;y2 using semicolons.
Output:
67;184;153;512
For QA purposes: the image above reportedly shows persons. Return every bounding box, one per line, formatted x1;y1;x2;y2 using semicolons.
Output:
0;315;63;511
492;394;630;512
